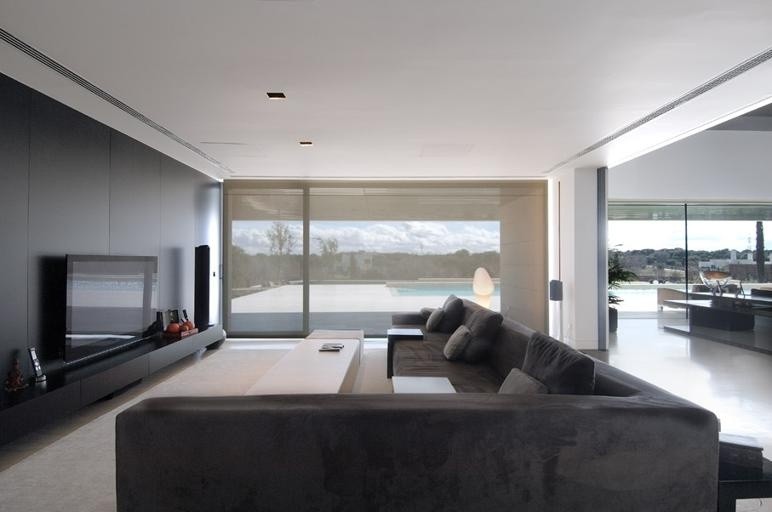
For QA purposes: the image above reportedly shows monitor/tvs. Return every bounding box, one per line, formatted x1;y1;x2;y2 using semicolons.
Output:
63;253;160;363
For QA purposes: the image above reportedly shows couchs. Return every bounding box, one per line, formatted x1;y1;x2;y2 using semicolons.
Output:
116;299;719;512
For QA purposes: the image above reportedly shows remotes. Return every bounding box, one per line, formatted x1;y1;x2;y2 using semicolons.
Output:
323;345;344;348
319;348;340;351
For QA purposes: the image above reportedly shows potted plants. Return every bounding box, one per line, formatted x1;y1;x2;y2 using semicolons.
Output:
609;243;639;333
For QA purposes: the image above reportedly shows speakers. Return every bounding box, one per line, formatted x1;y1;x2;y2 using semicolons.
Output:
194;245;210;333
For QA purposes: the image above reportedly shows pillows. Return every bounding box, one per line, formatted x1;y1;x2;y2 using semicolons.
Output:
426;293;597;395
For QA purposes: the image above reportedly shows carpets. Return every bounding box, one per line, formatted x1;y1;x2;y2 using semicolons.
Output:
1;349;392;512
